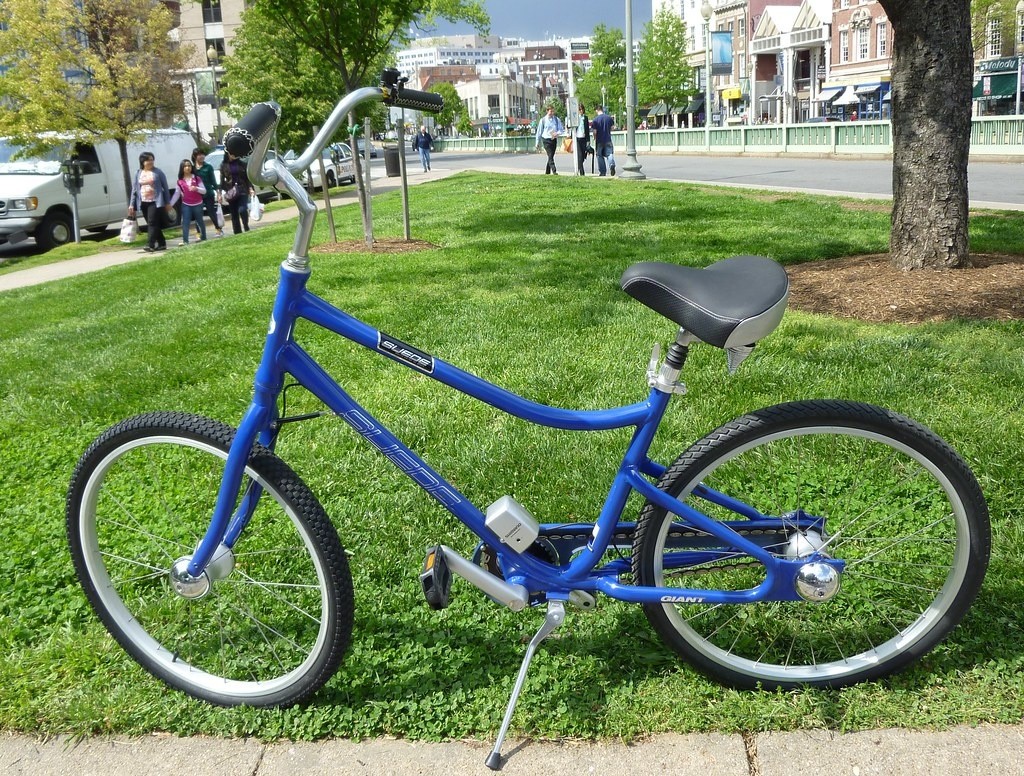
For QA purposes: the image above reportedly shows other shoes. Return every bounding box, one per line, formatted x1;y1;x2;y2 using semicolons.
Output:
179;242;188;245
213;229;223;238
143;246;154;252
156;245;166;250
599;173;606;176
195;238;203;242
610;164;615;176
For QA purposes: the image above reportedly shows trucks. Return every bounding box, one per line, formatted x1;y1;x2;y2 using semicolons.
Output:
0;130;201;252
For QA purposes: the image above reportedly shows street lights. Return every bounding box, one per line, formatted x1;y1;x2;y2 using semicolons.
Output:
498;63;509;136
206;44;223;145
602;84;606;112
701;1;714;126
618;96;624;130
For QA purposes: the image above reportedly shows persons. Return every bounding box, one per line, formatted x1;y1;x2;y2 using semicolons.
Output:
591;104;615;176
567;103;590;176
414;126;434;172
128;148;255;252
535;105;563;175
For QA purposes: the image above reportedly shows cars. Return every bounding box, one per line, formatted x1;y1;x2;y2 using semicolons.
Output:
203;150;287;213
803;115;843;123
344;139;378;160
284;141;367;191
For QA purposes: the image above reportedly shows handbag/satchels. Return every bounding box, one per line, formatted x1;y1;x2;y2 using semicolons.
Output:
215;203;225;228
585;143;594;155
119;211;138;243
225;185;240;203
561;139;573;153
250;194;265;221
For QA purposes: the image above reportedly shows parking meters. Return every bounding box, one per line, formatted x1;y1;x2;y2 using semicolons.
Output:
330;145;340;187
63;159;84;243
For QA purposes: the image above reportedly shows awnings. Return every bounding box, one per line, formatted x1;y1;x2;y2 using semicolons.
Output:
853;85;880;94
972;73;1018;101
812;90;840;101
647;100;704;117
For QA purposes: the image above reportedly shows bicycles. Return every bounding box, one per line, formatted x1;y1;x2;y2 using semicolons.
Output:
63;65;993;773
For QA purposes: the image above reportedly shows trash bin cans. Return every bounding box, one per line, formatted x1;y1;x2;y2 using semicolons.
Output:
382;144;400;177
